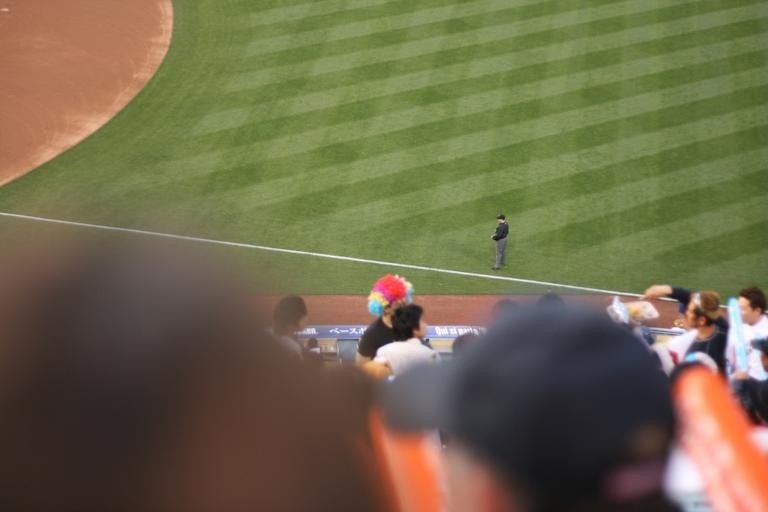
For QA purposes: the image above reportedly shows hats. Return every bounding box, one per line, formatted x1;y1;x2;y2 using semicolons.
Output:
750;336;768;351
689;289;731;329
373;296;675;507
495;214;505;220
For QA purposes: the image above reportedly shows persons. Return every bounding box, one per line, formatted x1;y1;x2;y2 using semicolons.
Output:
260;273;768;512
491;214;510;270
